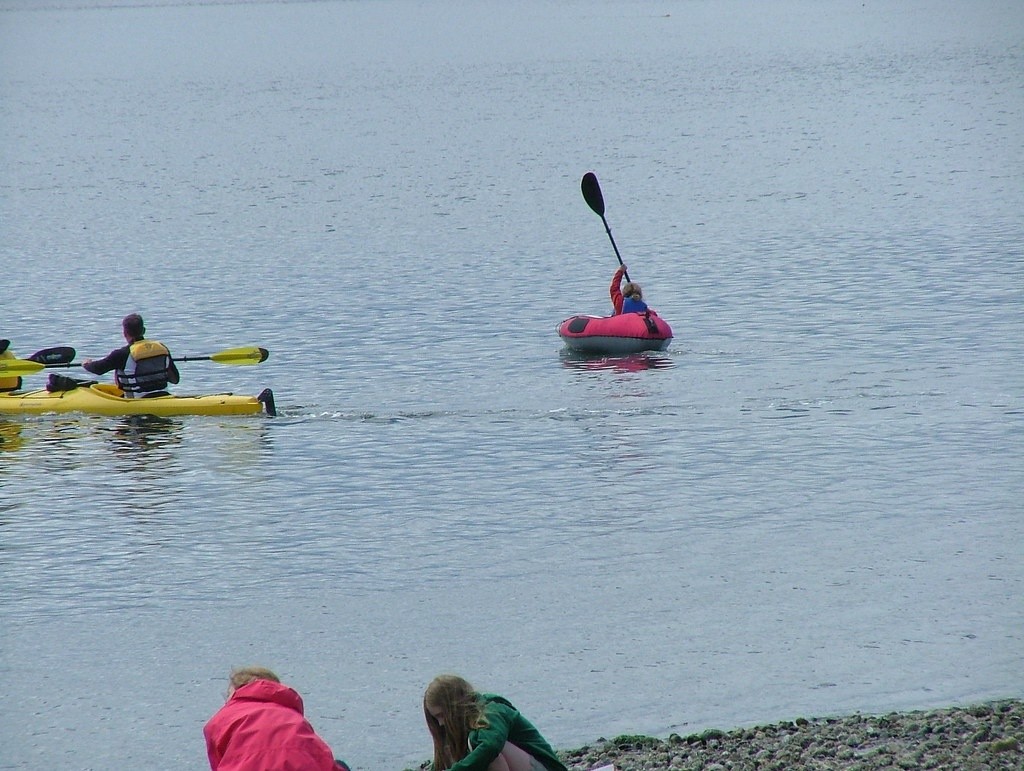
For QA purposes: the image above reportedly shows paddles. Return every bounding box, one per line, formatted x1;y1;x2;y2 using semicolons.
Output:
0;346;271;379
581;173;631;283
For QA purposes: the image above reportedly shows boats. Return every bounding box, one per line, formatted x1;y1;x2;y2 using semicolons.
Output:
555;312;674;353
0;351;277;416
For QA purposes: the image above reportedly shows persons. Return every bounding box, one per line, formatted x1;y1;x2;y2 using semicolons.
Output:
203;667;350;771
423;674;568;771
82;314;179;399
609;264;648;316
